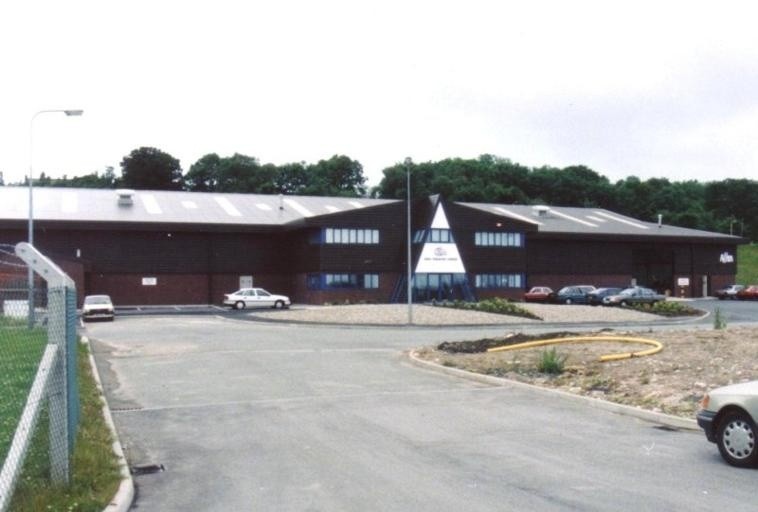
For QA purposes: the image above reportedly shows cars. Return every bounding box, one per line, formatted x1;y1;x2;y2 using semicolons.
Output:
695;381;758;467
525;284;664;308
737;285;758;299
715;284;744;299
223;288;292;311
83;294;116;323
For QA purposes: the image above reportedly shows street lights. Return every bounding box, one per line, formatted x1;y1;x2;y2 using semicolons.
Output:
24;106;85;327
730;218;738;236
402;157;415;325
